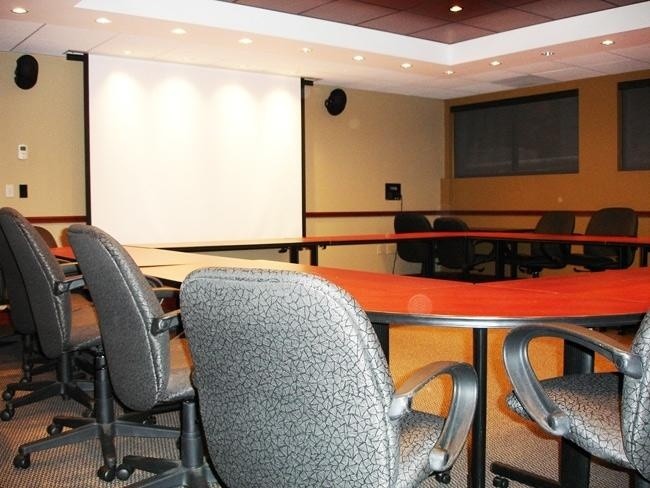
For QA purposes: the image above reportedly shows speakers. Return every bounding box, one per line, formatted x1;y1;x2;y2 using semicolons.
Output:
326;88;347;116
14;54;39;90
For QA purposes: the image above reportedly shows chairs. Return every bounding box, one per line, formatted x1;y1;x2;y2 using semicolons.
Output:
178;267;477;487
393;212;437;276
2;262;98;420
492;313;649;487
67;224;224;488
2;207;180;481
432;217;497;282
518;213;574;279
563;207;637;273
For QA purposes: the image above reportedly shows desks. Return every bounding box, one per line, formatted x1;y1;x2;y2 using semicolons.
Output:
45;229;649;488
